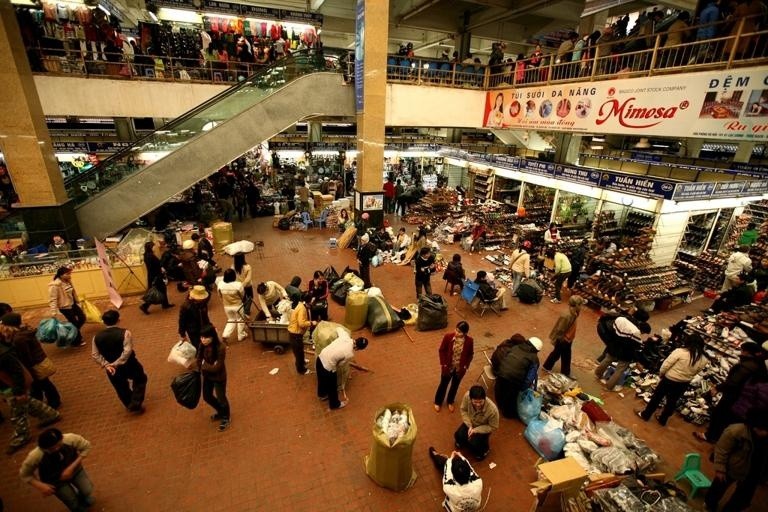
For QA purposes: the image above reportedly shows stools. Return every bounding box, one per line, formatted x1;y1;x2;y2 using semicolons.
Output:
443;279;464;297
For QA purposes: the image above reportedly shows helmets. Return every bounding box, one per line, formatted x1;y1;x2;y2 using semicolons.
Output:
529;336;543;352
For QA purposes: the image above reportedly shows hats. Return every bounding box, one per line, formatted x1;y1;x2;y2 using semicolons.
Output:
190;285;208;301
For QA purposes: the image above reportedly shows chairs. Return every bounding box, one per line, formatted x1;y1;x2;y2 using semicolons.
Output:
477;350;496;393
672;453;712;499
302;212;313;232
389;57;483;85
314;210;327;231
462;278;505;318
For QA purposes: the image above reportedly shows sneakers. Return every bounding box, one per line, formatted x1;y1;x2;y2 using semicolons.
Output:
401;186;552;250
598;298;768;440
488;197;693;313
534;367;661;479
673;200;768;297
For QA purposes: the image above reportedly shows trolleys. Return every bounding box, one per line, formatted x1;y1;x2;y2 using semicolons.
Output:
236;297;295;355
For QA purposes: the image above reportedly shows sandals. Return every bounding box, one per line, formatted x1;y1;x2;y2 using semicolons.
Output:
211;414;231;432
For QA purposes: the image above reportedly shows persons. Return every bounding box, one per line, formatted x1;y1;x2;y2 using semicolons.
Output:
196;327;231;431
14;1;291;82
399;0;768;84
429;447;482;512
1;164;17;216
698;218;768;316
597;305;708;427
91;310;147;414
486;93;504;127
510;101;520;115
541;295;588;379
384;160;438;215
316;327;368;410
59;151;134;204
693;342;768;510
490;334;543;419
2;236;74;263
50;267;86;347
435;320;474;412
188;148;355;221
1;303;61;456
19;429;94;510
140;232;328;375
454;386;499;461
337;209;617;310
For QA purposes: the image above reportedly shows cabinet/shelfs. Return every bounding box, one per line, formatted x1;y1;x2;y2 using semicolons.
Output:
535;184;766;311
400;187;558;252
